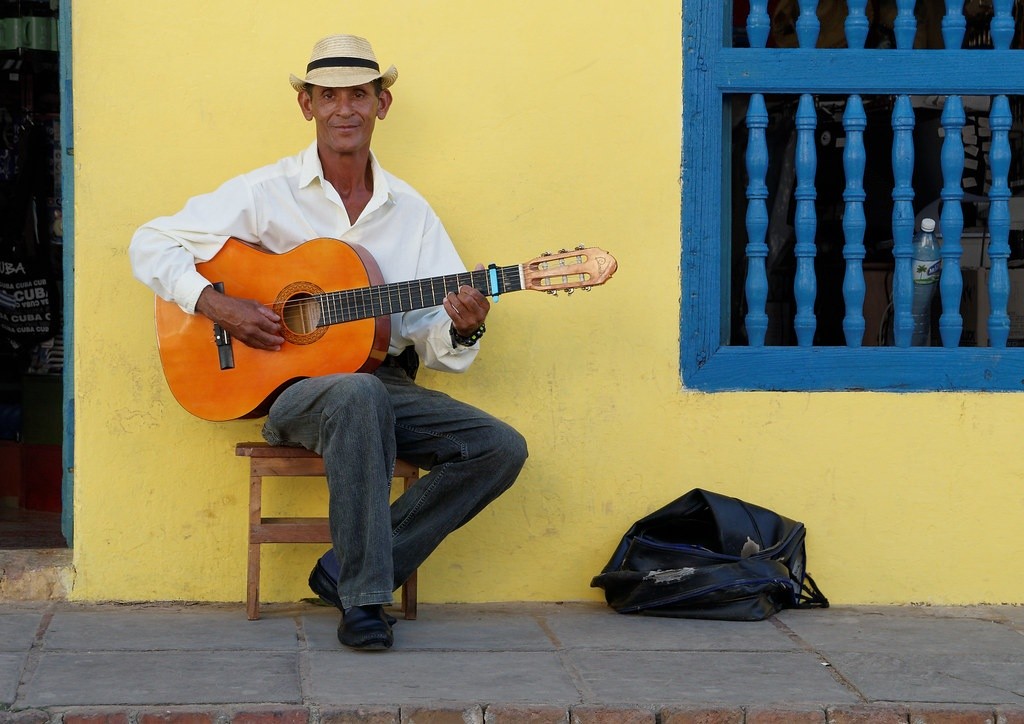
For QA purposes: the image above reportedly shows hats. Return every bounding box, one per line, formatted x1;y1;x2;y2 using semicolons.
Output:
289;35;399;92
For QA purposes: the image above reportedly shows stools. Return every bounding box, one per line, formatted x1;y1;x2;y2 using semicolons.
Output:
235;444;420;623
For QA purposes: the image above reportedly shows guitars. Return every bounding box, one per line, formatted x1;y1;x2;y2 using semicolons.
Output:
152;233;620;425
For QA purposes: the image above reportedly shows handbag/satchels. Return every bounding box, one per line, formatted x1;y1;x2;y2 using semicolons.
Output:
592;489;829;621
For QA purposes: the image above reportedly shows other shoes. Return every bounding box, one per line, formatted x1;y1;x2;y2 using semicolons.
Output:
309;548;394;649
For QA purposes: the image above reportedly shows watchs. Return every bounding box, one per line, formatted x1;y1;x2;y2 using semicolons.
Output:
450;323;485;348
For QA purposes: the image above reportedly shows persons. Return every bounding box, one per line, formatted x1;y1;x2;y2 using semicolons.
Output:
128;32;527;651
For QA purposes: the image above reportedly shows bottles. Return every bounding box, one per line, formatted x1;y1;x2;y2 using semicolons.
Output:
913;218;941;346
